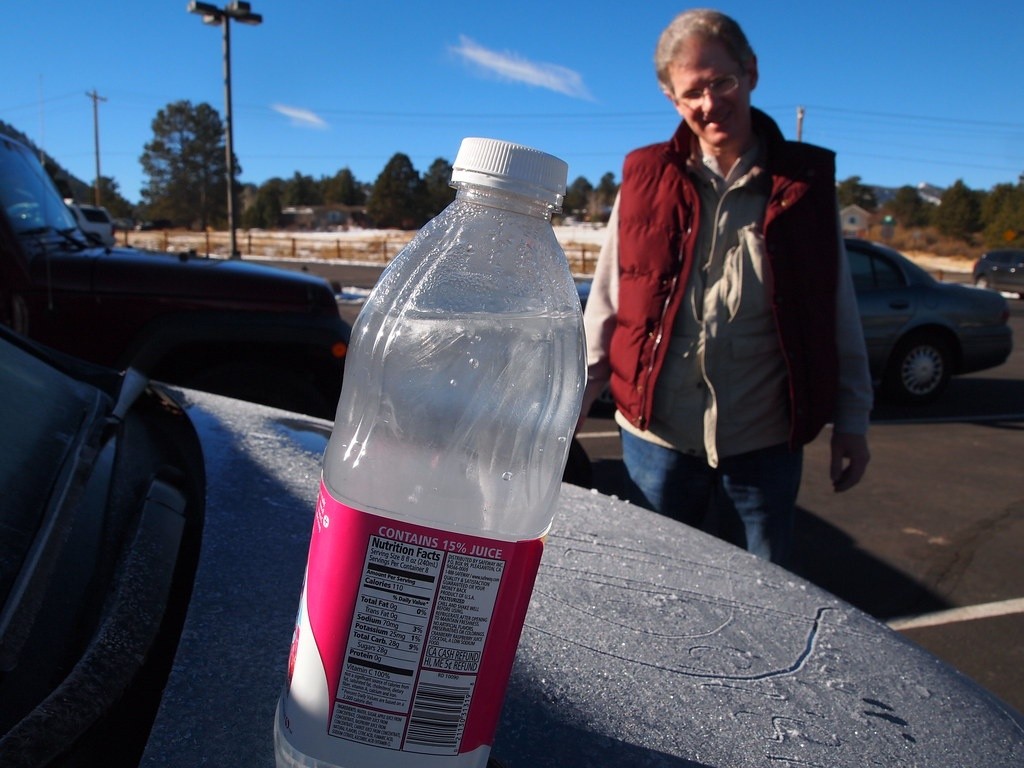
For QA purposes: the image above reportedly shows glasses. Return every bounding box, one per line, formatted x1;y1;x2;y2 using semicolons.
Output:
670;66;749;108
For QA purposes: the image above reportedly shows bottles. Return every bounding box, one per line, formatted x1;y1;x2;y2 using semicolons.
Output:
273;134;589;768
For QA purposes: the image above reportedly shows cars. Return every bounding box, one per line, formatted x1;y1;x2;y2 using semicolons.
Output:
973;249;1024;302
3;251;355;417
564;237;1014;420
0;344;1024;768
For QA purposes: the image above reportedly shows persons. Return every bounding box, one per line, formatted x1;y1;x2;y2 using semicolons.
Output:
580;7;876;572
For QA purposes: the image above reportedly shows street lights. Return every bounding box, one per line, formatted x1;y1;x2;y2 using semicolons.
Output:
186;0;264;261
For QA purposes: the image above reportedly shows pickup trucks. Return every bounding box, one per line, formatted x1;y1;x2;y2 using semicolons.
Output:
7;199;117;250
